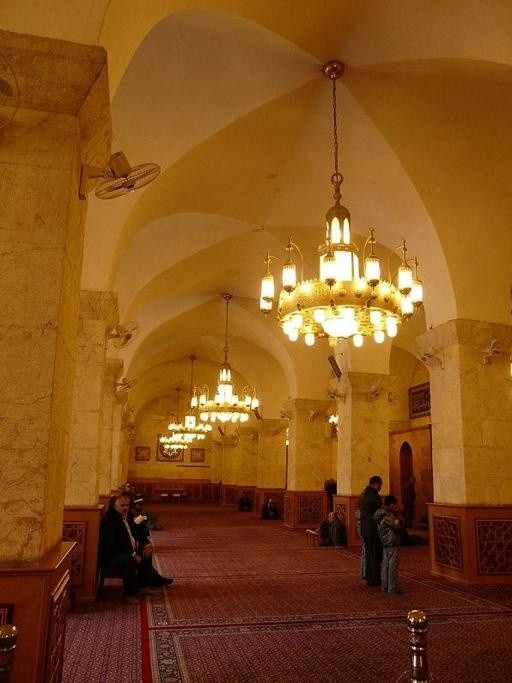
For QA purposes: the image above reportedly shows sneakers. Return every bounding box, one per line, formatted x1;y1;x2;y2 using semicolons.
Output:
360;574;403;594
152;575;173;587
124;595;139;605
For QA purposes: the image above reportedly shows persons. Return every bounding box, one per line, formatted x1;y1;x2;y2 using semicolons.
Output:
100;482;173;586
405;476;416;527
315;512;346;548
356;476;411;594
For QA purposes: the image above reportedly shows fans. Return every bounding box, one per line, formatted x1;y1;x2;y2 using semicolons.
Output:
77;149;163;202
104;317;140;393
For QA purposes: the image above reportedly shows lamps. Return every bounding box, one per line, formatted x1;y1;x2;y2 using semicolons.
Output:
159;292;260;456
251;57;425;351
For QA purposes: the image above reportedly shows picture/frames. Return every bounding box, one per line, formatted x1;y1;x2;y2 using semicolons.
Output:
136;433;205;462
